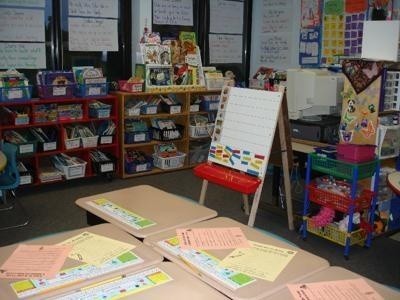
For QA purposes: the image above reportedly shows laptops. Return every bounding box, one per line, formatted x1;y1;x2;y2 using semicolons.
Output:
338;20;400;63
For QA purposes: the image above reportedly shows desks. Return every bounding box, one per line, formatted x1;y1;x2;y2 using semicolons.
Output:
142;216;332;299
31;261;227;299
74;184;219;242
263;265;400;300
0;223;165;299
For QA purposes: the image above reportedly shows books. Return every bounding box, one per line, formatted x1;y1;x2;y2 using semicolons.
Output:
0;31;231;176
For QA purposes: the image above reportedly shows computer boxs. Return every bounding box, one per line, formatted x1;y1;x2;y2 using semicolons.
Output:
289;115;341;142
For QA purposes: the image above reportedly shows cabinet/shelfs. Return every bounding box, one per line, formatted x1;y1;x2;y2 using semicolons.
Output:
302;147;377;258
121;89;221;178
0;92;120;187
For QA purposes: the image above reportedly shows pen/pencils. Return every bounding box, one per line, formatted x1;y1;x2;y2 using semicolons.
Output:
77;274;126;291
178;255;201;276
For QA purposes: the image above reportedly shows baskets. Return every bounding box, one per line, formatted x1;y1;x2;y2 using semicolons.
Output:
117;78;143;91
91;151;120;177
305;150;379;179
74;82;110;98
152;151;186;171
151;123;185;140
190;123;215;137
1;86;34;103
304;214;369;247
14;161;63;186
124;130;152;144
303;175;378;214
0;103;114;149
53;156;87;180
124;101;218;116
37;84;74;99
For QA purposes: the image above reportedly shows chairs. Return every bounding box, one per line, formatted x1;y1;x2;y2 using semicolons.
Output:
0;141;32;230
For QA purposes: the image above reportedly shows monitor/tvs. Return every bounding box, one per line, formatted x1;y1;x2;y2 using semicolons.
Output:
287;69;344;122
